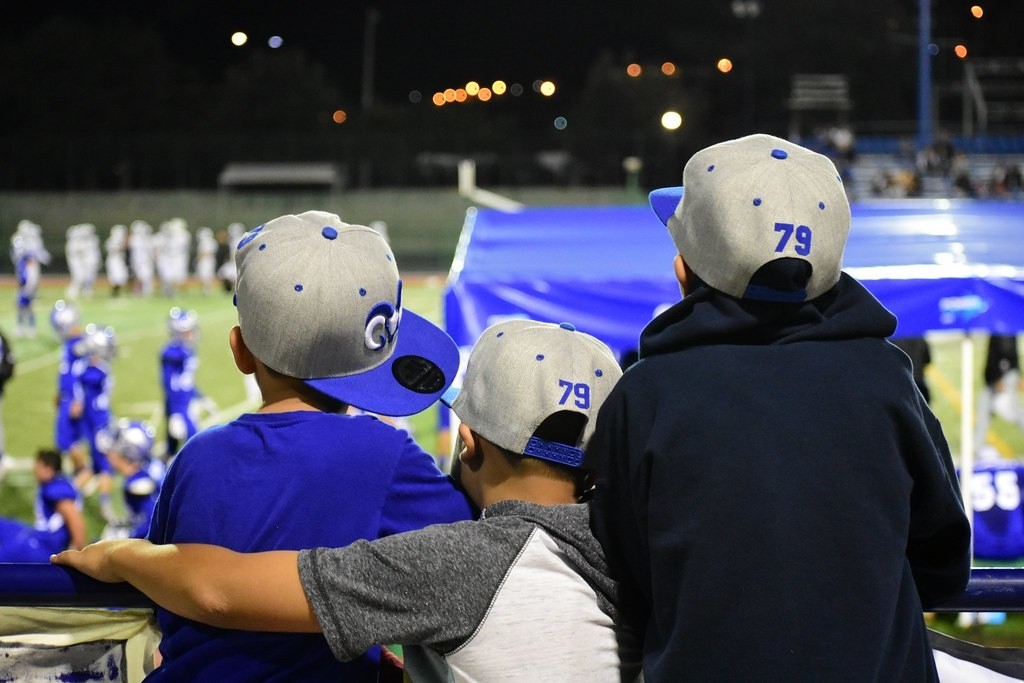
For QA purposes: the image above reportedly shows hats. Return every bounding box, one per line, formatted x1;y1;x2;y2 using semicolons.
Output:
648;134;851;310
235;210;460;419
443;318;623;470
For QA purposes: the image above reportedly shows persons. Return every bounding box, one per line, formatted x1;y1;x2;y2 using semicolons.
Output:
584;133;971;683
49;319;624;683
34;308;216;552
7;217;248;333
0;331;54;563
145;209;475;683
974;333;1024;454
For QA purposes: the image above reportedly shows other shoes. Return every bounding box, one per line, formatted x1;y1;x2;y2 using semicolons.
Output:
15;324;39;341
99;502;116;524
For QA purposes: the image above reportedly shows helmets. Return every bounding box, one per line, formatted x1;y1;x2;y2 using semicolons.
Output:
74;330;117;357
51;304;80;330
96;418;156;460
167;311;198;334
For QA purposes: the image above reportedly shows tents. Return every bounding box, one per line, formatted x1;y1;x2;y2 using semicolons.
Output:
442;195;1024;596
